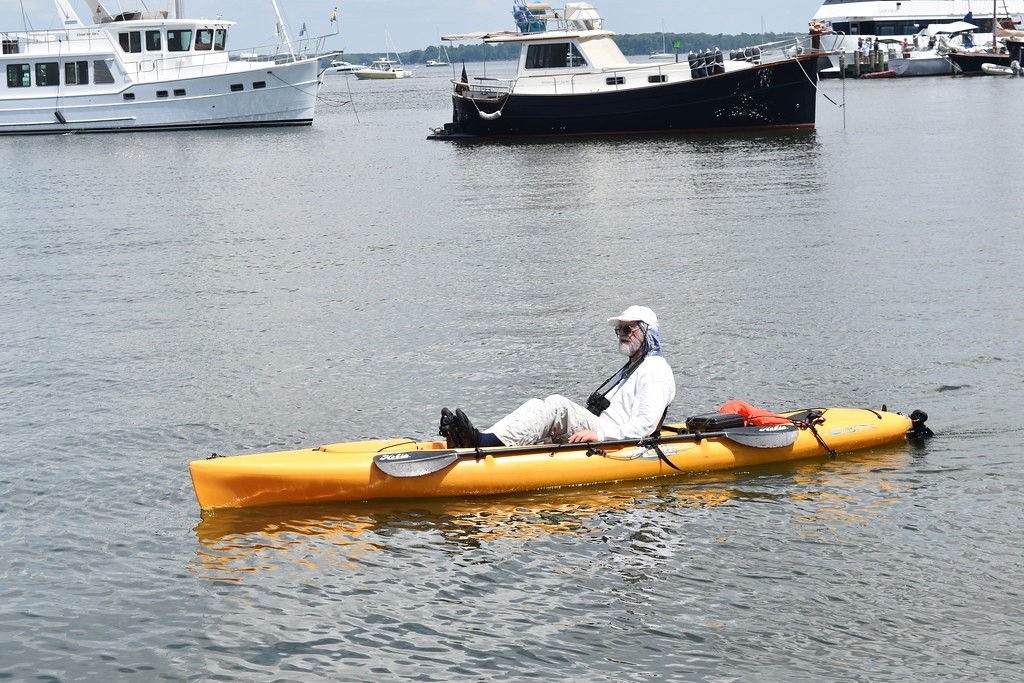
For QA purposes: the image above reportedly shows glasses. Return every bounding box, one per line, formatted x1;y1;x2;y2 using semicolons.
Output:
614;324;640;336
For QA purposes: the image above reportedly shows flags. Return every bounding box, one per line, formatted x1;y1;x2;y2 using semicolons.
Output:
299;23;306;36
329;10;337;25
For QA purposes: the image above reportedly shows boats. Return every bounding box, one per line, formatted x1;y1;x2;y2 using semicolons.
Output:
324;24;412;79
938;0;1024;74
787;0;1024;79
0;0;344;135
426;0;847;139
189;404;935;512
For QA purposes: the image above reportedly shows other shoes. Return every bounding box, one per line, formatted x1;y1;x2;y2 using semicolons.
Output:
441;408;463;447
456;409;480;447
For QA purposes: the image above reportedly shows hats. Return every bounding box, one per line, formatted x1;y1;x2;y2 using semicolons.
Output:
608;306;659;333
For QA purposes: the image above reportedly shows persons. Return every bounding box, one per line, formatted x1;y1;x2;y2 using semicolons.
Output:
874;37;879;57
858;37;862;47
861;38;870;63
902;38;908;48
441;306;676;449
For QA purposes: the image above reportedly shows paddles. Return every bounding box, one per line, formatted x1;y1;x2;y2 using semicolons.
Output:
371;422;800;480
